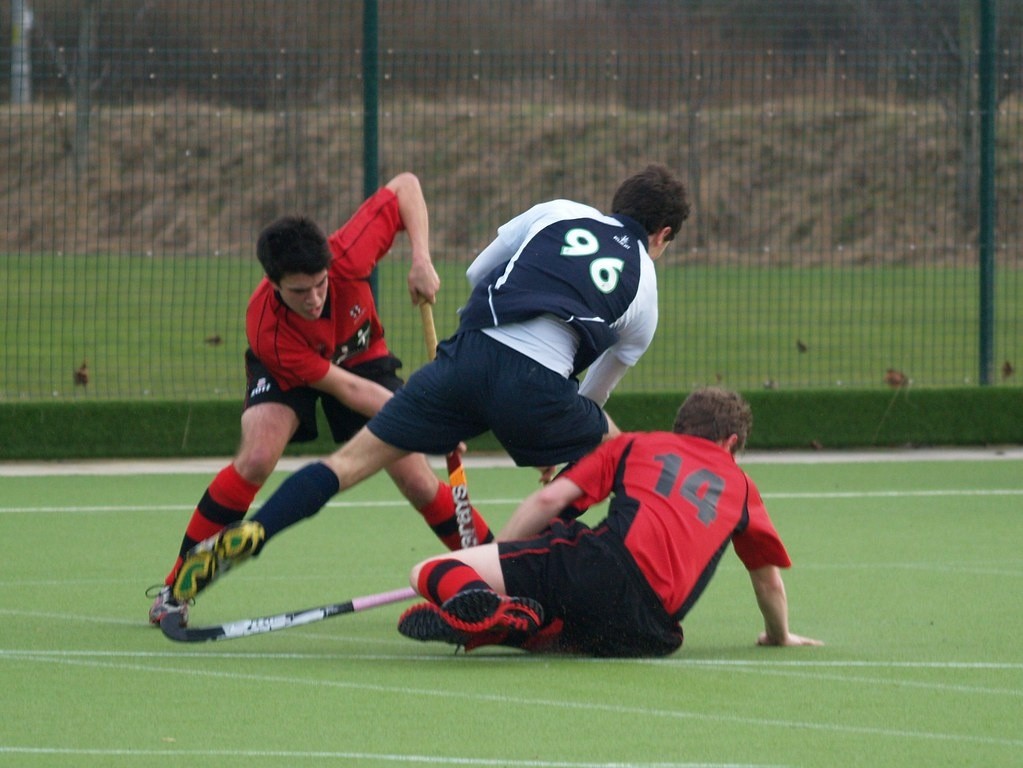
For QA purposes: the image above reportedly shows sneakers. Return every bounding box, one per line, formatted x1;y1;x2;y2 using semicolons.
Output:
399;603;471;654
439;589;544;634
145;582;196;628
171;519;266;606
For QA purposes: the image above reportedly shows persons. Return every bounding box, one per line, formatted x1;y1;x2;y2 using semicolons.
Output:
172;161;690;604
145;171;496;628
398;386;824;657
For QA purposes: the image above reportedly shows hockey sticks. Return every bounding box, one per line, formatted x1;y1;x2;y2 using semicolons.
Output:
418;289;478;550
159;586;421;643
